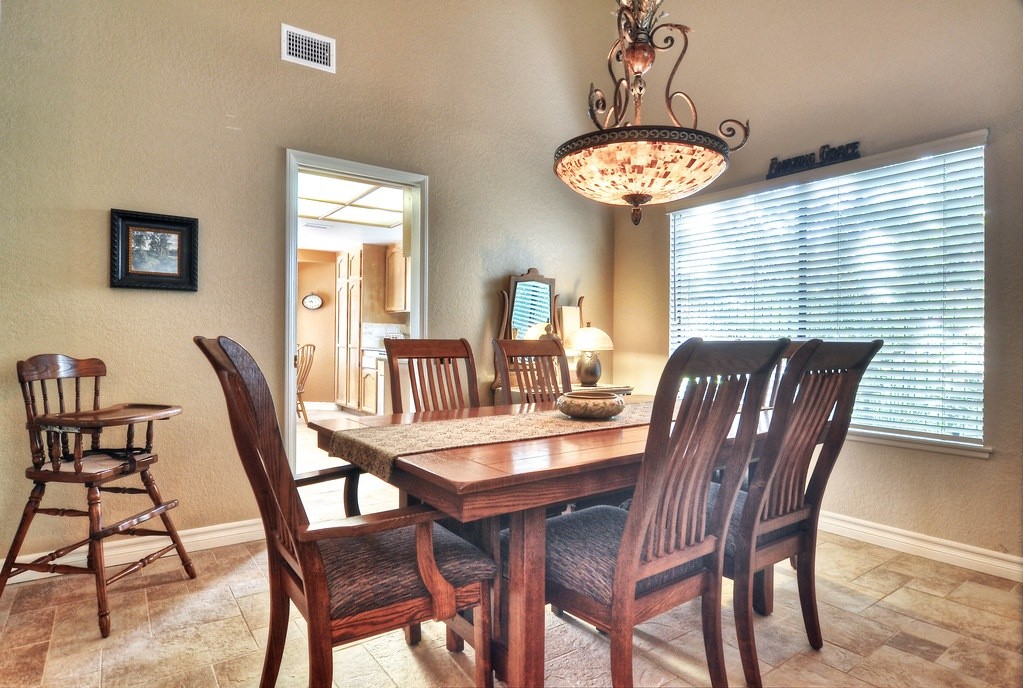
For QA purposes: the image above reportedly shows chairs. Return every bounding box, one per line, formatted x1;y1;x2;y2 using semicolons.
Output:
295;344;315;425
491;337;616;640
0;352;199;640
620;337;886;688
489;338;792;688
194;336;498;688
384;337;569;653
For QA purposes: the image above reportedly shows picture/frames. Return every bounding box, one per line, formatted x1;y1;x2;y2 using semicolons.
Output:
109;208;199;293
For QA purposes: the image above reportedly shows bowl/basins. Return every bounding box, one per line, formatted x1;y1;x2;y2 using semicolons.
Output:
557;390;626;419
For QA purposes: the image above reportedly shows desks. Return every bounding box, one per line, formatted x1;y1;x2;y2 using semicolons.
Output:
307;392;833;688
494;384;634;406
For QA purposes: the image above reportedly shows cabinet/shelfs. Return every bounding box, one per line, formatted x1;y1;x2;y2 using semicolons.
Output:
334;243;409;418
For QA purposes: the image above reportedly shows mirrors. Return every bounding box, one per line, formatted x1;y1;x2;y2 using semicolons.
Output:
492;266;556;389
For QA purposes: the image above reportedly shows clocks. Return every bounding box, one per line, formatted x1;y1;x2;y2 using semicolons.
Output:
303;295;322;309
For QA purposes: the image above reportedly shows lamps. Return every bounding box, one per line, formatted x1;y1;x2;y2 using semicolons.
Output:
553;0;753;225
564;322;616;388
